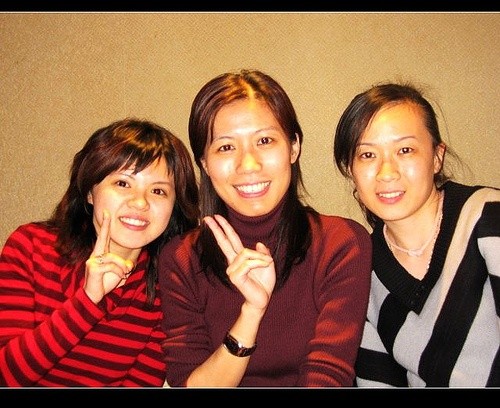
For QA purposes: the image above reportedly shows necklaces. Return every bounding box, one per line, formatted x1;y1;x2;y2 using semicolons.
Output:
382;193;440;259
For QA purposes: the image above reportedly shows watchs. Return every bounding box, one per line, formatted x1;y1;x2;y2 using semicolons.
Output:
221;330;257;356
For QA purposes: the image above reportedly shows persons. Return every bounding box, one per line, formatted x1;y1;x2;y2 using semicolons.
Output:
157;69;377;391
0;117;204;388
332;82;498;389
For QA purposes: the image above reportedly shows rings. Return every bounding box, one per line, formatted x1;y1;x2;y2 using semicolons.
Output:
96;255;104;266
